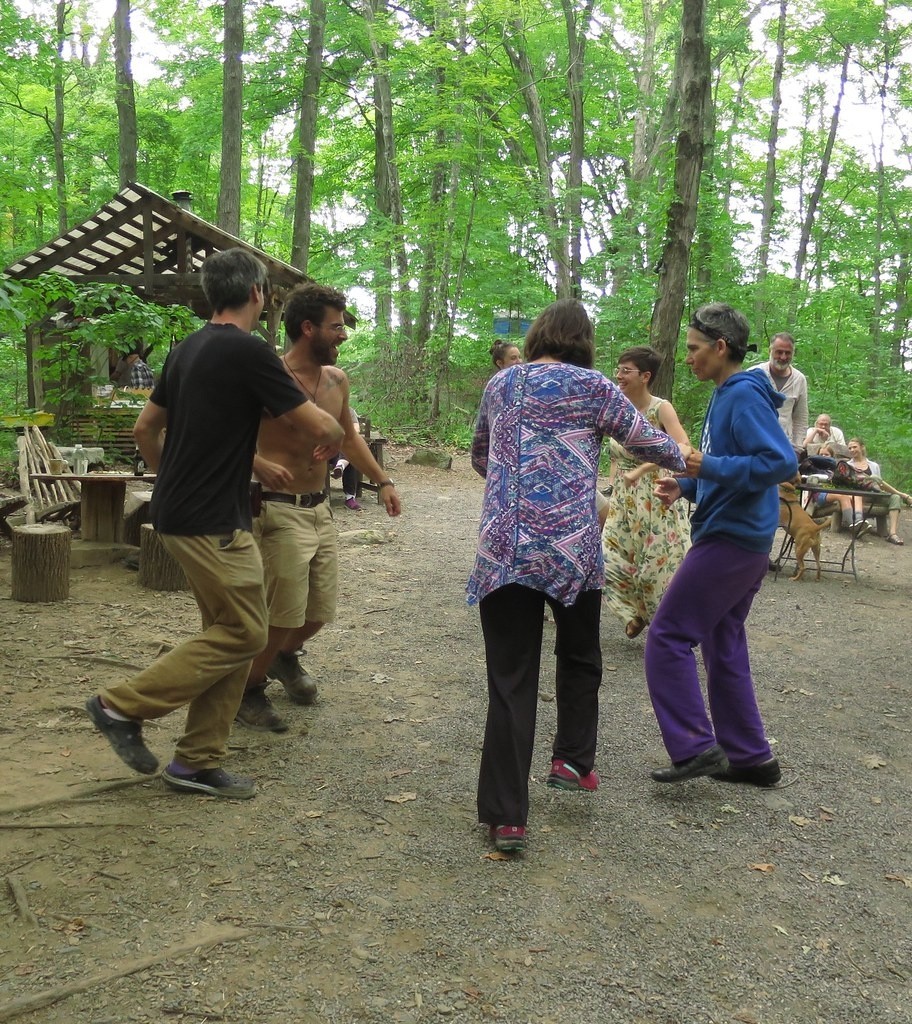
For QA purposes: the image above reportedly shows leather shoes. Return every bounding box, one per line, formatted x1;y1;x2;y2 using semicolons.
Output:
651;746;729;783
709;756;782;786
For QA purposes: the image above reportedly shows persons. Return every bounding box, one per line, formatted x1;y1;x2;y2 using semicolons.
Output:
331;406;360;509
460;295;809;850
803;414;912;544
86;248;402;800
122;350;154;390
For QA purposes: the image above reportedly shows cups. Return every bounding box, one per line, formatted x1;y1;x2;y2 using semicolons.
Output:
50;458;63;475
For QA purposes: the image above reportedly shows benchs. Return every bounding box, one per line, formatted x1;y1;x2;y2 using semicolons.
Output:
828;483;891;533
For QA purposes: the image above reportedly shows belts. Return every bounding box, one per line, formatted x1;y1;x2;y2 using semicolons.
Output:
261;489;327;508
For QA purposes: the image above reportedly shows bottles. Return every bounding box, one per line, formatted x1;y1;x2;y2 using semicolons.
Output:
72;443;87;476
133;449;144;476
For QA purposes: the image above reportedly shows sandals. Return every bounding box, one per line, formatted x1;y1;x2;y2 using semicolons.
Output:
885;532;904;545
903;493;912;506
626;616;646;638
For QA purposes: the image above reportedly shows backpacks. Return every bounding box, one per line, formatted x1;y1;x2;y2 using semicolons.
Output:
799;456;884;492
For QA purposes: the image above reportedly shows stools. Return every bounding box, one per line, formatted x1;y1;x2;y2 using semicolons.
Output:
139;523;192;593
10;523;72;604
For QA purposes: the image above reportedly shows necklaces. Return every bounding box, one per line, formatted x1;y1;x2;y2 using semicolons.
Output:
284;355;322;405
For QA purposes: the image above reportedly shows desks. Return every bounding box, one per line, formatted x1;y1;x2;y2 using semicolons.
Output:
29;471;157;544
773;482;891;584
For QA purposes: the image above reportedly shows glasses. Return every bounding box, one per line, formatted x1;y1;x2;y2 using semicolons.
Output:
614;367;640;374
321;324;347;331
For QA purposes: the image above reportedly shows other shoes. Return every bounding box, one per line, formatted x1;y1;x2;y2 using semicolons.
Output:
848;520;873;539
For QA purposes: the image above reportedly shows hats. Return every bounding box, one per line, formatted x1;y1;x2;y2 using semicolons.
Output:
122;348;140;362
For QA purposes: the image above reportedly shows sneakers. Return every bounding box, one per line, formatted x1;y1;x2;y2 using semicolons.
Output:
768;559;783;572
548;760;599;792
489;823;526;853
344;496;361;511
237;681;290;732
330;465;343;479
272;650;323;703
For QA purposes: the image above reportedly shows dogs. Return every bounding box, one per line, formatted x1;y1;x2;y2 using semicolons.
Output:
777;470;831;581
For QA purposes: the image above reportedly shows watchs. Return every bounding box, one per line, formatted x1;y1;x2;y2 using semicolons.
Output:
378;479;395;489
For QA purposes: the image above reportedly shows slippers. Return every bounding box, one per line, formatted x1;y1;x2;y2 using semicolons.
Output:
161;758;257;799
85;694;159;775
